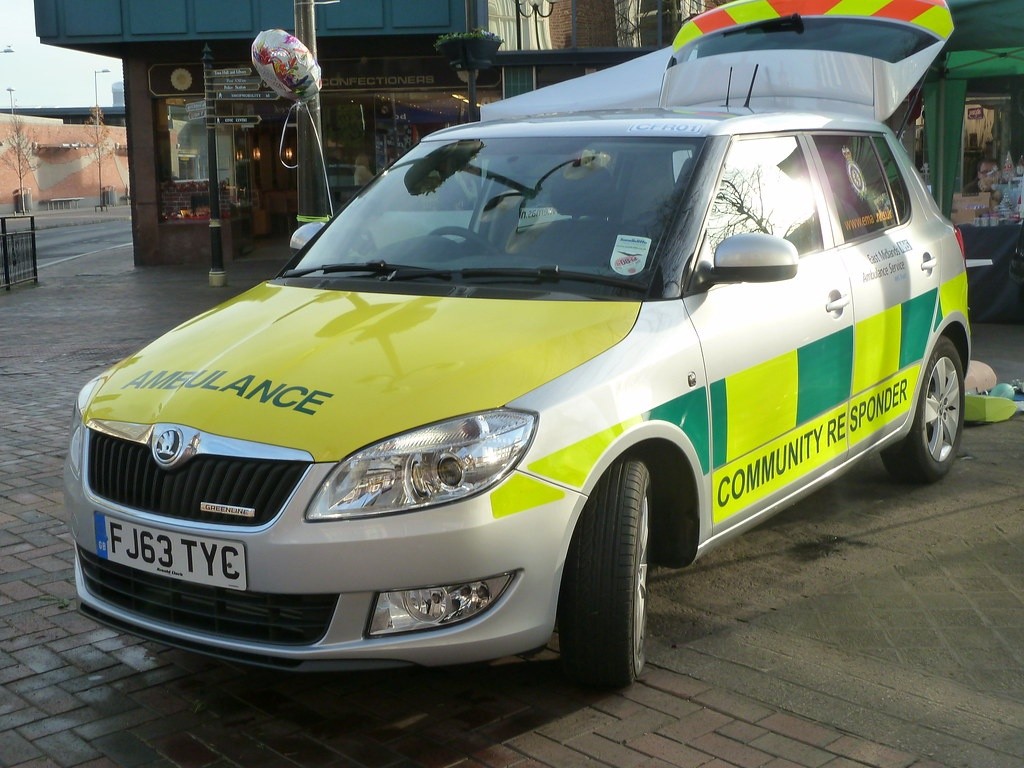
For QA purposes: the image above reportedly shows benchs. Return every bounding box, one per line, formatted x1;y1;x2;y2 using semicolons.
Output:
47;197;84;211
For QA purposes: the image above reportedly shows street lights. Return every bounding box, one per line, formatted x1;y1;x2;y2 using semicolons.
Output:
93;69;112;211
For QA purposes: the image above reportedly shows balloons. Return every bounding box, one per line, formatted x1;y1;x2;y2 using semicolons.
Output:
250;26;323;105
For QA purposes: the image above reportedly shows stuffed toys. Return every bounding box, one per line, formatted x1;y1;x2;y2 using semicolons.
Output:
977;177;1001;207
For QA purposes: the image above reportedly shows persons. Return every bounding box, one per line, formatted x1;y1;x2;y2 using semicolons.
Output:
352;154;375;186
970;159;1006;195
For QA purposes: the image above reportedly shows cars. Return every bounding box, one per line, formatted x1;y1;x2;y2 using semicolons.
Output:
63;11;975;694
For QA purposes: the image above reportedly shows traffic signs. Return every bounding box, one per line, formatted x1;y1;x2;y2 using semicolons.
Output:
203;66;281;126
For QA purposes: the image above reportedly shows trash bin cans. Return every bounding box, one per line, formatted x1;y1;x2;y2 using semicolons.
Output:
100;186;118;208
13;186;33;213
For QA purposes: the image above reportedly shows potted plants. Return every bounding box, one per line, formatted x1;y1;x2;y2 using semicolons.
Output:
435;30;508;60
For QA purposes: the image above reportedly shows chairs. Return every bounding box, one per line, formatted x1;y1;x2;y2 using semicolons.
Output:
251;190;298;237
523;166;617;264
